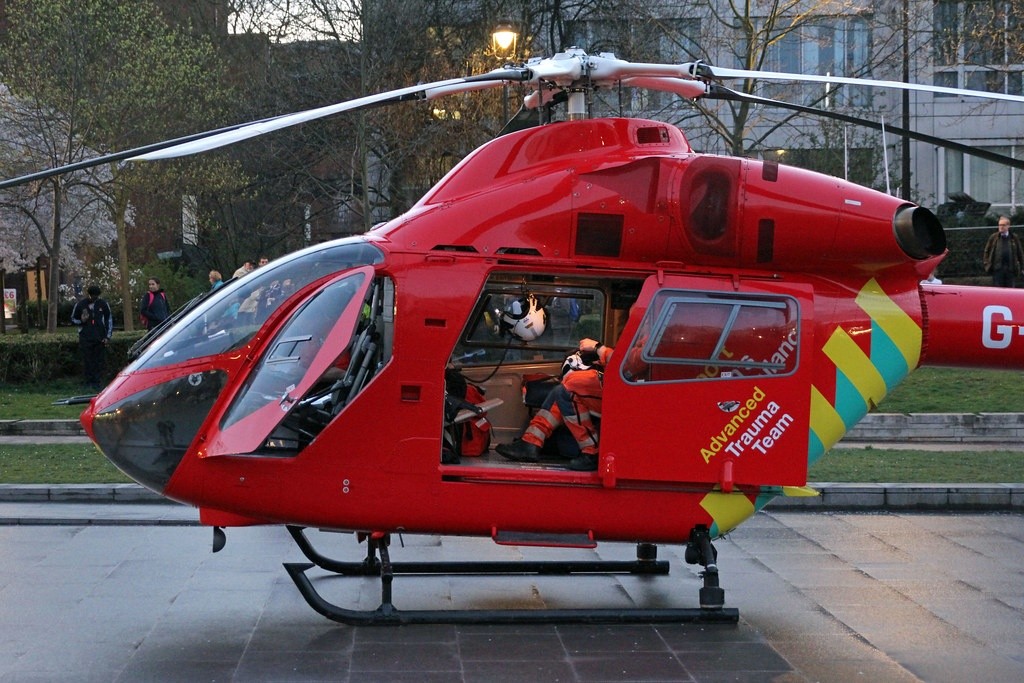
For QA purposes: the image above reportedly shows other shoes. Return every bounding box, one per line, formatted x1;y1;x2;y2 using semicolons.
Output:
568;452;598;471
495;437;543;462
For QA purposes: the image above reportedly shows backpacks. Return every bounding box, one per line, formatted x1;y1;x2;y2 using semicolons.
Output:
140;290;165;325
459;380;492;458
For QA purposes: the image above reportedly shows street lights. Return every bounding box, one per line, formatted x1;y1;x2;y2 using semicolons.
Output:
489;16;519;128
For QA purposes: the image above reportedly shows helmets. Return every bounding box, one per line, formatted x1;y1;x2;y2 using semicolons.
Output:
561;347;601;384
499;292;547;341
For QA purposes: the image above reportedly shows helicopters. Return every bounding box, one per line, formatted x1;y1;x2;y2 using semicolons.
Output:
0;44;1024;629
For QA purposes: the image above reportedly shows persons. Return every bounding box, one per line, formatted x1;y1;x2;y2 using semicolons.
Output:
983;216;1024;288
71;285;113;388
920;270;941;284
496;302;652;470
140;276;172;338
208;255;282;326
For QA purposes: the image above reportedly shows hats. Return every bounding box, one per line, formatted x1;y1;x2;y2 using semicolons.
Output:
88;286;101;295
244;256;258;265
